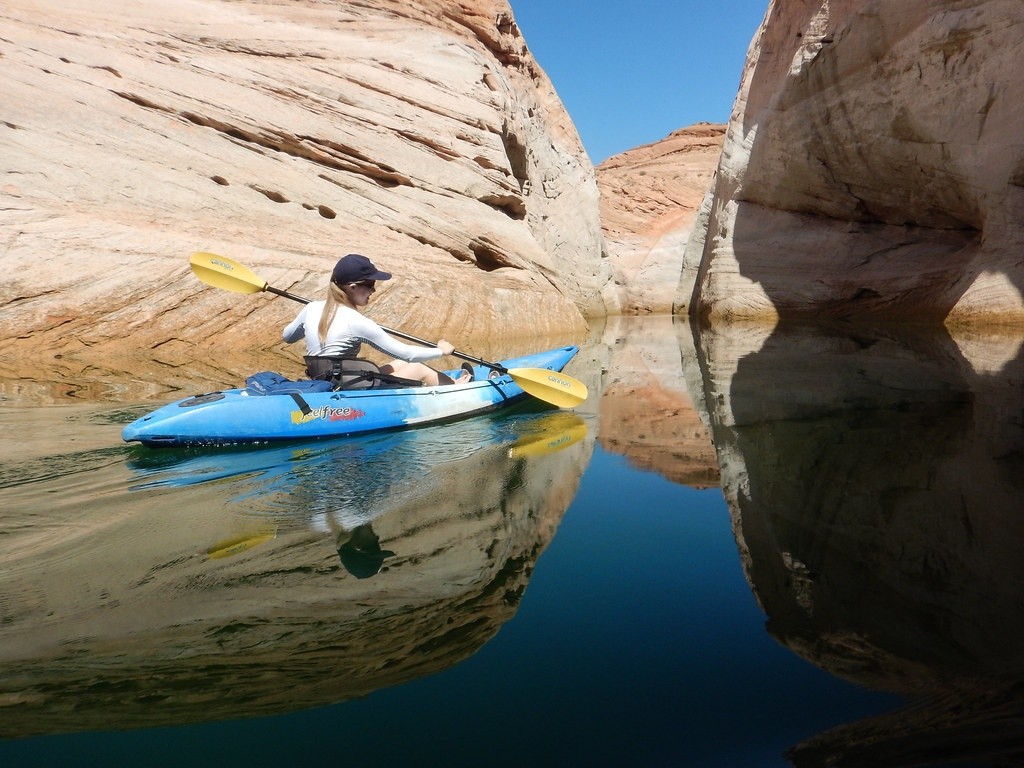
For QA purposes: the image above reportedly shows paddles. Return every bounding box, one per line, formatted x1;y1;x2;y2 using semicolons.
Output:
187;249;590;410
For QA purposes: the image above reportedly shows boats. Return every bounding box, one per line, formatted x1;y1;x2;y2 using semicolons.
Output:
122;344;583;445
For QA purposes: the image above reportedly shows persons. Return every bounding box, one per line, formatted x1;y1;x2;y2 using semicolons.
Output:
312;462;397;579
283;254;471;388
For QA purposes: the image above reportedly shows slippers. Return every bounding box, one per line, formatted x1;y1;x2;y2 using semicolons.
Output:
488;363;504;379
461;362;474;382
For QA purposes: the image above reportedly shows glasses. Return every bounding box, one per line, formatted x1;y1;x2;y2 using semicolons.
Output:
350;282;374;291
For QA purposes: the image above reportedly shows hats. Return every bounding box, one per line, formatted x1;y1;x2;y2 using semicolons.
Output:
330;254;392;283
339;550;397;579
